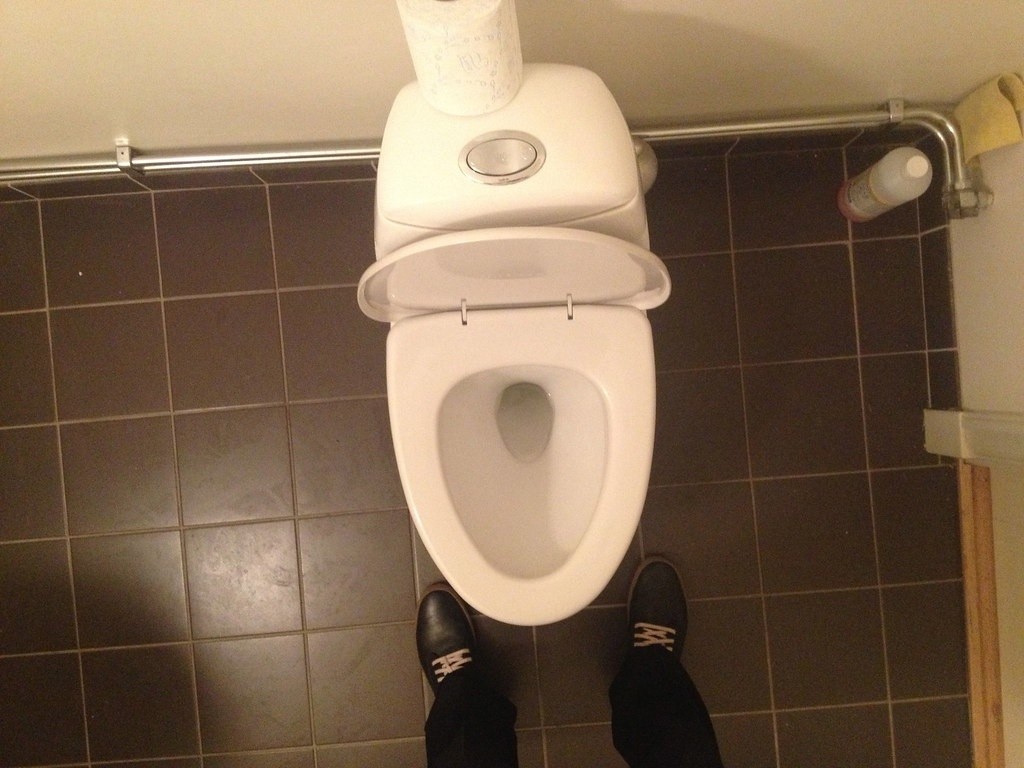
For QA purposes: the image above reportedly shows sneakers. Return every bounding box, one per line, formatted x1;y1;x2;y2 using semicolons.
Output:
625;556;687;664
415;583;480;699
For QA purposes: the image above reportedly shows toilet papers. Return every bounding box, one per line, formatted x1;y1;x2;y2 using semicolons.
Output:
394;2;525;112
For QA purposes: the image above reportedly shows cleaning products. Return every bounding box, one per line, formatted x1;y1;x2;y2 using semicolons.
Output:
834;146;934;223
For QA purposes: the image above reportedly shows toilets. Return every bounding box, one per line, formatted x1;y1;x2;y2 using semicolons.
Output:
350;59;676;631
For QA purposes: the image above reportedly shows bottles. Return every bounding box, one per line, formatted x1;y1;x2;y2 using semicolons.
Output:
837;147;932;222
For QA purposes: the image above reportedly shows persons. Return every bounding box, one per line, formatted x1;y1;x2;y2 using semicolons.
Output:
414;556;725;768
